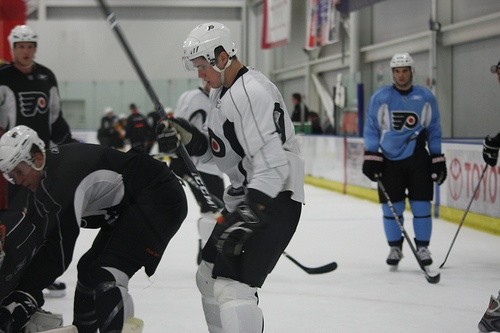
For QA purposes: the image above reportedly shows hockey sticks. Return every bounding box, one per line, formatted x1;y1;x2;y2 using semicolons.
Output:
439;162;489;269
376;176;441;283
98;0;226;225
176;169;338;276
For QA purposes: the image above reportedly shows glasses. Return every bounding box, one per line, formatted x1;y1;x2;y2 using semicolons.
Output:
13;45;35;50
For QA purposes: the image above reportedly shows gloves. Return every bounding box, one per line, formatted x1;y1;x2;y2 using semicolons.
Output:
482;134;499;167
213;200;266;260
361;150;384;182
430;153;447;185
155;115;206;158
0;276;45;333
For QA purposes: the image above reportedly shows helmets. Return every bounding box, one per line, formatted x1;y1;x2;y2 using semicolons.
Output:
7;24;38;59
183;22;237;65
389;53;415;84
0;125;46;181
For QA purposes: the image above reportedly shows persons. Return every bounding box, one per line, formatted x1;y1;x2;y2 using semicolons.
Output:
157;22;304;333
97;103;172;157
0;24;73;298
171;80;224;265
307;112;322;135
0;125;188;333
290;94;309;122
362;52;447;283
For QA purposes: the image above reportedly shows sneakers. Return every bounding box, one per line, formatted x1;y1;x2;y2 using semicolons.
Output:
42;281;66;298
478;294;500;333
415;238;440;283
387;236;404;271
